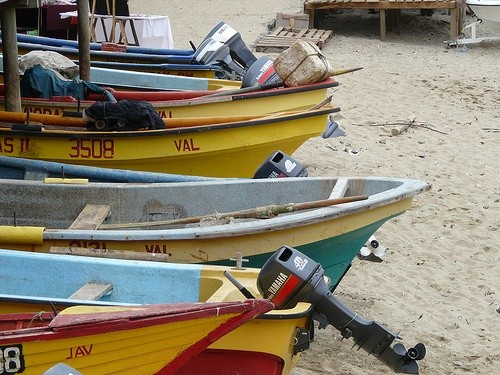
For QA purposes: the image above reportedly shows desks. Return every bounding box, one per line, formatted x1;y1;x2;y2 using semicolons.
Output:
302;0;467;41
76;13;173;50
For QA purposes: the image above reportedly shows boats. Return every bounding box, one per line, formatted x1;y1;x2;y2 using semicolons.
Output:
0;176;432;295
0;245;426;375
0;21;345;179
